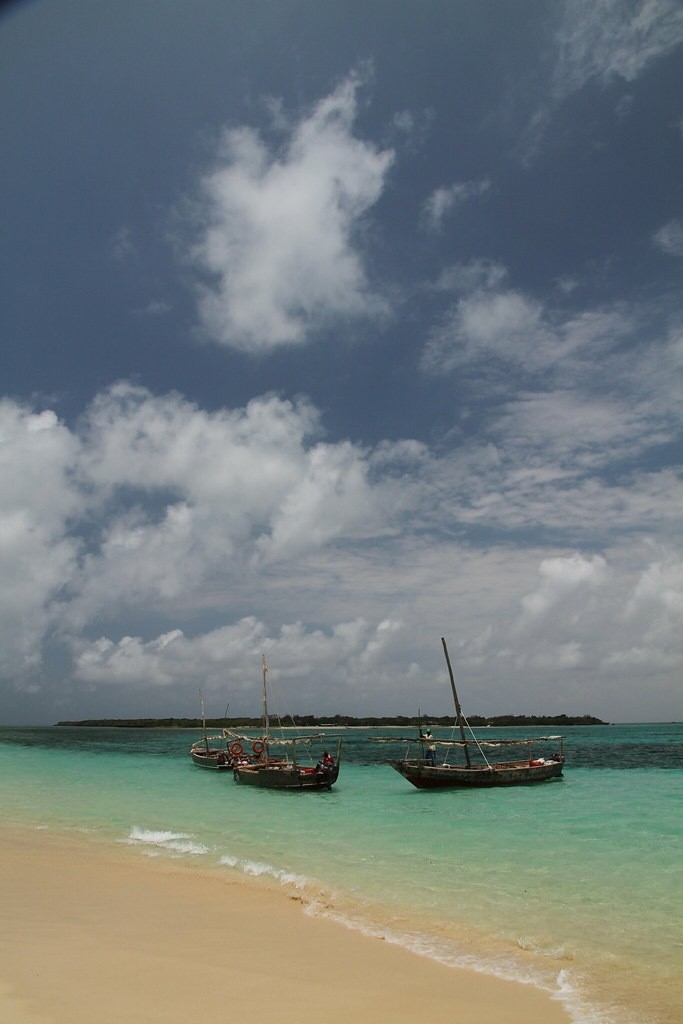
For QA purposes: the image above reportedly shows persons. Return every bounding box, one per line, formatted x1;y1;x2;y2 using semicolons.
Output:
319;751;334;772
419;728;436;767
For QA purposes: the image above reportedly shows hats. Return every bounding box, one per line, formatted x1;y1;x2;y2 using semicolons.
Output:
427;728;431;733
322;752;328;757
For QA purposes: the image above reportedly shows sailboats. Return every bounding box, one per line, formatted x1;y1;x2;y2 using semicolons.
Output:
229;655;341;788
191;690;257;770
386;639;565;788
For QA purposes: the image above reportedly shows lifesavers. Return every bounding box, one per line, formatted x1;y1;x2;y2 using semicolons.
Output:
231;742;243;755
253;742;265;753
